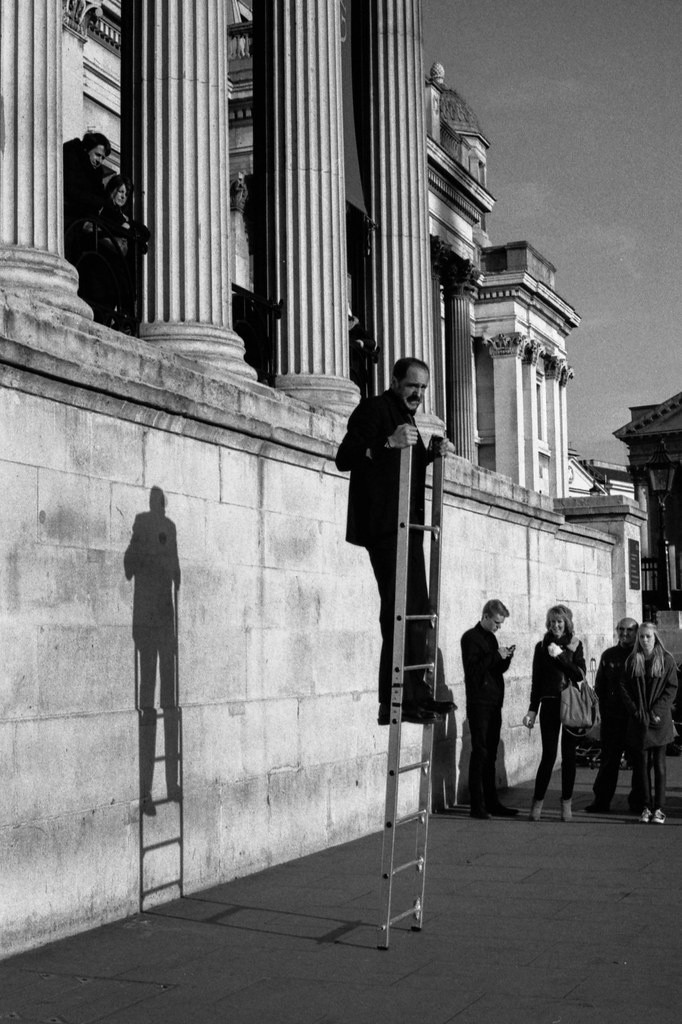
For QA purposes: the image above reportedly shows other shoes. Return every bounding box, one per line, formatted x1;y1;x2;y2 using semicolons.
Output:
377;696;457;725
584;805;611;812
469;810;490;819
491;806;520;817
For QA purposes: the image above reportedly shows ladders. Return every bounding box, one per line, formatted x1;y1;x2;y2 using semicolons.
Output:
373;427;450;953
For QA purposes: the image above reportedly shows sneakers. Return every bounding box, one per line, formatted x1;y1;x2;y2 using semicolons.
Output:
651;809;666;824
638;808;653;823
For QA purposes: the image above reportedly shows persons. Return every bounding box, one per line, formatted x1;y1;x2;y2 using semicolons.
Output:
63;133;151;335
523;605;587;821
585;618;677;823
461;600;519;820
336;357;456;725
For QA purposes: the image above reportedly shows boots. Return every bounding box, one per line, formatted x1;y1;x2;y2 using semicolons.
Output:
560;799;574;823
529;799;544;821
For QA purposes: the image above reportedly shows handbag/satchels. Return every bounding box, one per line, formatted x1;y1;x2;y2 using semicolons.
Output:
560;666;602;738
